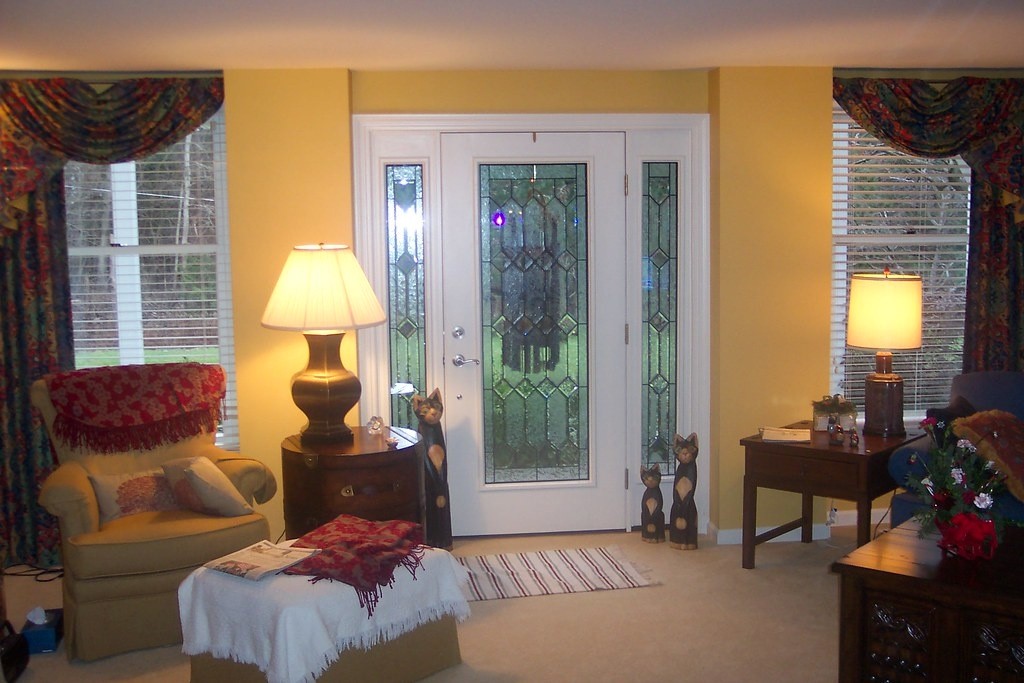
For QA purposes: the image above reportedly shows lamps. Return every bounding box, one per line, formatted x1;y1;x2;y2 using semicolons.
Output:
261;240;387;447
847;266;923;436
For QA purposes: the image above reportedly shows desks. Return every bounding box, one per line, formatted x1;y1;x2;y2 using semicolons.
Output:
739;419;927;568
281;427;427;545
829;513;1023;683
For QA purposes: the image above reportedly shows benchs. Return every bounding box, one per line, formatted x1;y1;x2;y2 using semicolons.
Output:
177;539;473;683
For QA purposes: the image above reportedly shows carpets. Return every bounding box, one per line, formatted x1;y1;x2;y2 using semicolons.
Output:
454;546;662;602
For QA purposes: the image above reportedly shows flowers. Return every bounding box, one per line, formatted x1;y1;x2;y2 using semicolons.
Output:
905;413;1024;543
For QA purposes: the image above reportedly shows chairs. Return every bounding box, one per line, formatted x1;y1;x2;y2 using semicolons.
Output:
29;361;276;663
892;372;1024;528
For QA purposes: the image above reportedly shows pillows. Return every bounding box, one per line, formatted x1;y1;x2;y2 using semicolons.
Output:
953;408;1024;501
925;397;977;447
89;467;180;526
160;458;254;517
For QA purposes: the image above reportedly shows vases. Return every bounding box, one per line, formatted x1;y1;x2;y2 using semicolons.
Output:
932;511;998;562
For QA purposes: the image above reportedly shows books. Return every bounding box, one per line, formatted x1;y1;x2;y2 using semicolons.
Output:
762;426;811;443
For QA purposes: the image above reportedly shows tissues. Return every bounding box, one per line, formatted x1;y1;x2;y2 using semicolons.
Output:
18;607;63;655
